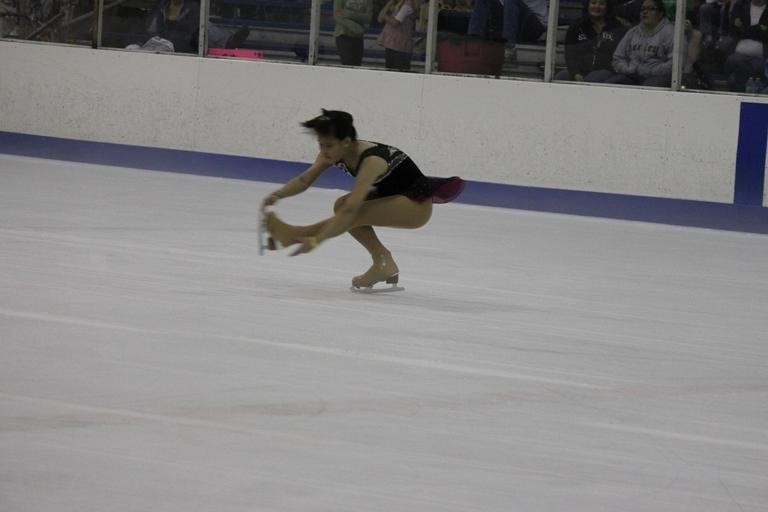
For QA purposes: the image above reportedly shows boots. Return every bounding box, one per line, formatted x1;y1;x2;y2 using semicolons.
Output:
353;253;399;287
267;211;307;250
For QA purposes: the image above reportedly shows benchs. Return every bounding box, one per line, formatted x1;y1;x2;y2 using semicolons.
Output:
209;0;768;97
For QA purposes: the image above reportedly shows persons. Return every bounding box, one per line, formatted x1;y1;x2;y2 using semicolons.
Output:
377;0;549;70
261;108;464;289
145;0;199;54
333;0;372;67
555;0;767;93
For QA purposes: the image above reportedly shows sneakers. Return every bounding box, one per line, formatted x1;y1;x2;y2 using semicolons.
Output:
226;27;249;49
503;50;517;68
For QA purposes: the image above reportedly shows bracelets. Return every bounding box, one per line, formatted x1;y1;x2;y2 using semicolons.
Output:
271;191;285;199
309;233;320;247
